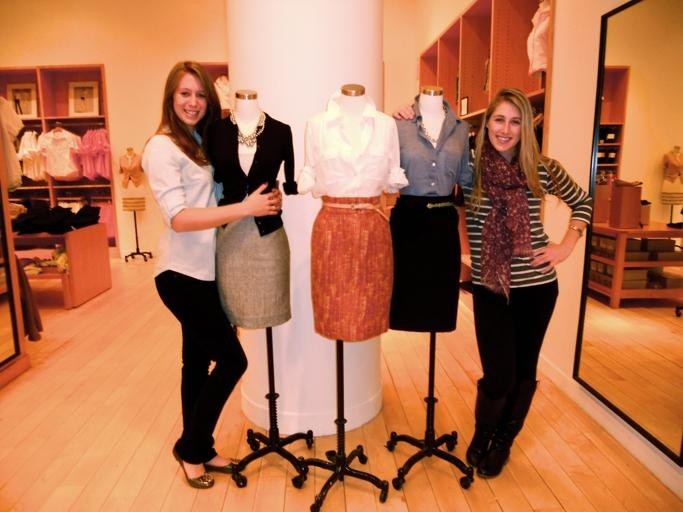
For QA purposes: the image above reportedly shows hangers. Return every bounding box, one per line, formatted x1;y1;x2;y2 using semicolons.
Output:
24;121;105;135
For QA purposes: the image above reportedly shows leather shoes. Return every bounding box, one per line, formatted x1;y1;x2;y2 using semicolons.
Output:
172;447;214;489
203;454;242;473
613;178;643;187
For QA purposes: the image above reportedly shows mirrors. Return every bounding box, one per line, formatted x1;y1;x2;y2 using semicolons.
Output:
0;190;22;378
571;1;682;473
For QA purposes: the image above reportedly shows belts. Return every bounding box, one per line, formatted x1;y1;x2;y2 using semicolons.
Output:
321;202;390;222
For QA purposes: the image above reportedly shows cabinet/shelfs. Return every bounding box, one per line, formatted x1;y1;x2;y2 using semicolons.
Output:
0;141;32;392
1;64;120;261
10;219;112;313
595;66;630;179
591;220;682;310
418;1;555;291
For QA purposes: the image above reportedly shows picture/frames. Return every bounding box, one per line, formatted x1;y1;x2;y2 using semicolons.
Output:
68;80;99;119
5;82;38;121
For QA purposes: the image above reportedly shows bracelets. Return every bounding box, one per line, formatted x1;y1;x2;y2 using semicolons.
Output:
568;224;583;237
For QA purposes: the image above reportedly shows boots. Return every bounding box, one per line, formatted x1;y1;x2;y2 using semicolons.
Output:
465;378;507;467
476;379;539;479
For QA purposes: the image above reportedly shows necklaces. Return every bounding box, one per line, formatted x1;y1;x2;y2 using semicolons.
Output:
424;108;445;142
230;111;268;146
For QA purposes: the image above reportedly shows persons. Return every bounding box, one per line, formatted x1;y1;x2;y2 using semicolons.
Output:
296;83;409;340
393;87;472;332
393;89;595;480
661;146;683;205
118;146;145;211
141;60;283;490
209;91;291;328
